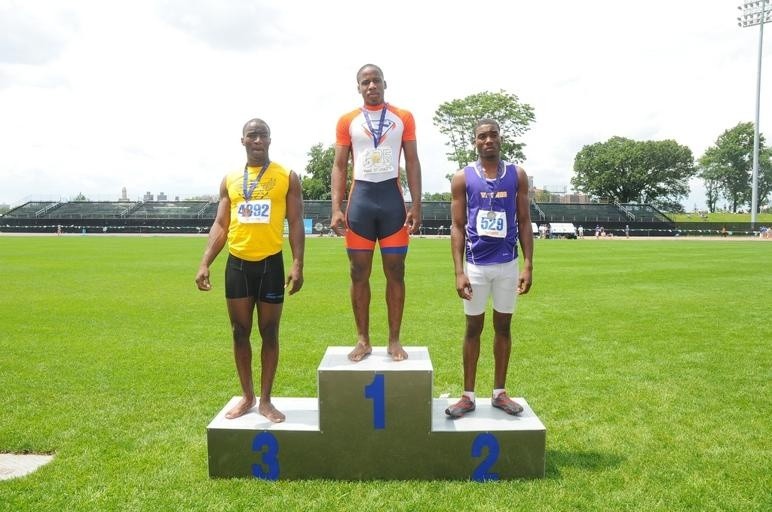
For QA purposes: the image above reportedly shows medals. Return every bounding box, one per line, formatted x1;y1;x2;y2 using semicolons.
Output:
241;208;252;217
371;151;381;162
487;210;497;222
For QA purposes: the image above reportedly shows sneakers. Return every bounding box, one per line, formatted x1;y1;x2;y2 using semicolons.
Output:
444;396;475;417
491;392;522;415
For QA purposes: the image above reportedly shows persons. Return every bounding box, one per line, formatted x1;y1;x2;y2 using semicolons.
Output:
759;224;772;239
624;224;630;239
328;63;422;362
721;226;727;237
418;223;423;237
443;117;534;419
537;221;606;240
436;224;444;238
194;118;305;424
